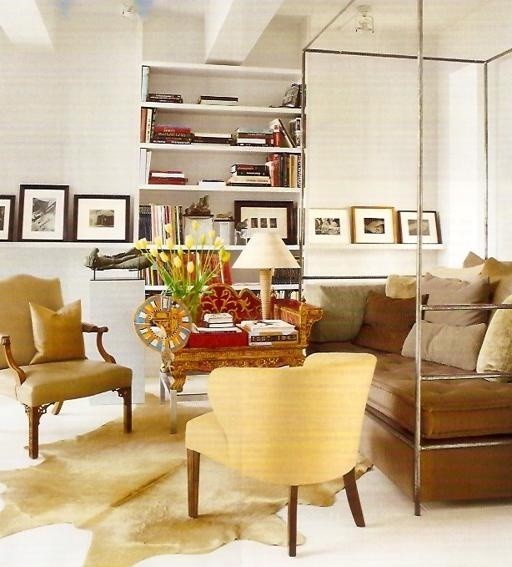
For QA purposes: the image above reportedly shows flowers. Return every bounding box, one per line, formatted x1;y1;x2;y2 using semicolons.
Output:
132;217;231;313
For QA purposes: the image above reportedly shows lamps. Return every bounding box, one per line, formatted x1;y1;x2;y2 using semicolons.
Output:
231;232;302;321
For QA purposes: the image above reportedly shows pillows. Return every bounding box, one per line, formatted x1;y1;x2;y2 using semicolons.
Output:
27;298;88;367
352;252;511;383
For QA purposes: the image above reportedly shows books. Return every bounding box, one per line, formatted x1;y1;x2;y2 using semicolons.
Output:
203;311;233;323
197;325;243;334
204;321;236;328
240;318;295;333
139;83;307;301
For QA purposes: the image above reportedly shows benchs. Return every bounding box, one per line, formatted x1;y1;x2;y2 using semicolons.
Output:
159;283;324;391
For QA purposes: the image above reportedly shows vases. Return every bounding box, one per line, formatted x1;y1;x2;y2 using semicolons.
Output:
172;292;202;325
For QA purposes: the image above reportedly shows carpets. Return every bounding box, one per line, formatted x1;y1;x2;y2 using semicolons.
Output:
0;390;378;567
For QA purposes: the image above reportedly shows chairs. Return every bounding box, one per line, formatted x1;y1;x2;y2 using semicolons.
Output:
184;350;380;556
2;272;134;461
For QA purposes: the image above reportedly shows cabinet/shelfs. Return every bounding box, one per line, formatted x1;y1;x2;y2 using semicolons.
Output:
131;61;302;293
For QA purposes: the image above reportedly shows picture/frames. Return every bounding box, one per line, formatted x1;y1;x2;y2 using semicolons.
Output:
234;198;297;246
304;204;440;246
0;183;130;244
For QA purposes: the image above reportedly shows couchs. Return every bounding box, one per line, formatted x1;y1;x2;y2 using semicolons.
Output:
310;280;511;514
